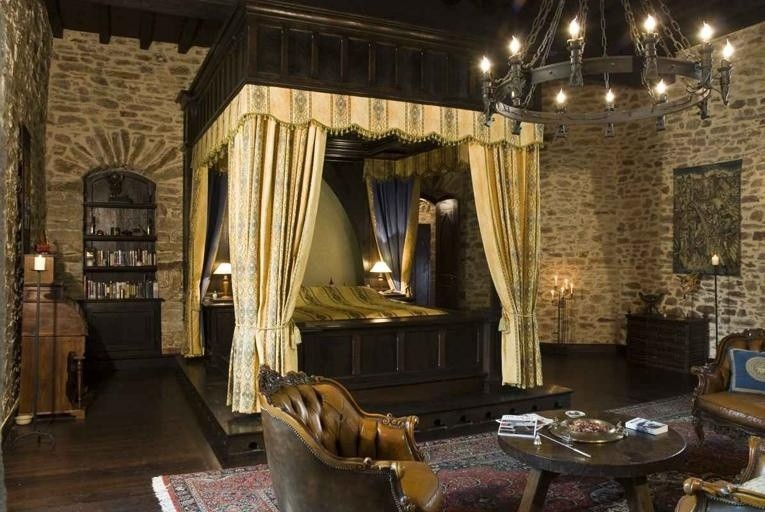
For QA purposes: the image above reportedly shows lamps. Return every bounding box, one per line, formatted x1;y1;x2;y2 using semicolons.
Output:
478;0;734;138
213;263;232;299
369;261;392;291
548;270;574;358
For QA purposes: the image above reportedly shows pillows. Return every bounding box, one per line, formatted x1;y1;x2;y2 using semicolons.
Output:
725;347;764;397
733;470;764;497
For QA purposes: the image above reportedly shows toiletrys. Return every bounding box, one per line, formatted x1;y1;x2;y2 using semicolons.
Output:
213;291;217;300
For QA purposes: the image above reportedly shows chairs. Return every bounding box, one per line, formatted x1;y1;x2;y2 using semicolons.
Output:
256;362;443;510
673;434;764;511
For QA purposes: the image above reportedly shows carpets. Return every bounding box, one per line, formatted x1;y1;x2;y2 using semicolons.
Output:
152;391;750;512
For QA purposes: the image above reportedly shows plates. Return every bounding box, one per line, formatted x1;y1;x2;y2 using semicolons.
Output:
565;410;585;418
551;417;625;444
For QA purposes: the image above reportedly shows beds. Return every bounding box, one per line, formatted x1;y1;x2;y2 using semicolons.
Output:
173;1;546;416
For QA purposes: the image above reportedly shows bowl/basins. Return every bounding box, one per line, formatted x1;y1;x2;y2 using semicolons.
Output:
15;415;32;425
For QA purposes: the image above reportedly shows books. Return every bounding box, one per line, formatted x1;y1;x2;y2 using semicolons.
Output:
83;247;159;299
625;417;668;435
495;412;553;438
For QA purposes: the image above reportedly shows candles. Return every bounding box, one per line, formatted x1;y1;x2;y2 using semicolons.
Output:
710;253;720;265
32;252;45;268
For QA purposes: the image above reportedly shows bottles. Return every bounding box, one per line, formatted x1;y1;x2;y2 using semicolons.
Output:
90;216;95;234
212;290;218;299
146;218;152;236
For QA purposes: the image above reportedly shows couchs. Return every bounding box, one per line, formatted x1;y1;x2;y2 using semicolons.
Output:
690;328;764;443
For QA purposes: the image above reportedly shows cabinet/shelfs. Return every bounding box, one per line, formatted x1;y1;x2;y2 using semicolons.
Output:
81;163;165;359
17;249;92;419
627;309;708;376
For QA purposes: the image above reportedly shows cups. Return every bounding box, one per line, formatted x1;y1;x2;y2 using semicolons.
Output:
111;227;120;236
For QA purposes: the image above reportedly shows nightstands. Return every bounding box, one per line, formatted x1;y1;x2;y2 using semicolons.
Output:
202;298;234;357
